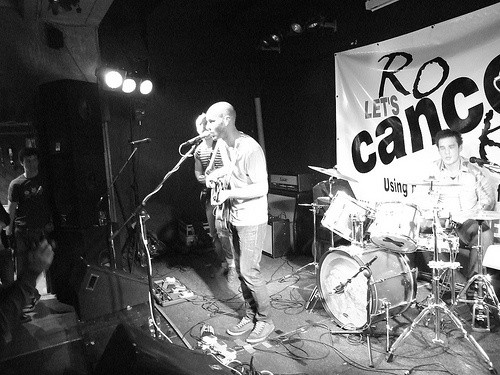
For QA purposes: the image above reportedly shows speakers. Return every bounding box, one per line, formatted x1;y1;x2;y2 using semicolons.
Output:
96;330;234;375
67;253;149;323
262;189;313;258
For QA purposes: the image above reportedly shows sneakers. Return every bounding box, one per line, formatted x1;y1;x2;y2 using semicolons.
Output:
246;321;276;342
227;317;255;335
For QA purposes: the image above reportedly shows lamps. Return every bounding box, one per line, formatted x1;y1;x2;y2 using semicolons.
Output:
94;66;154;99
271;19;309;41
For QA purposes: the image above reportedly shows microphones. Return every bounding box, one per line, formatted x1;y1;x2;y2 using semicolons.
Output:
130;137;148;143
183;131;211;146
470;157;498;166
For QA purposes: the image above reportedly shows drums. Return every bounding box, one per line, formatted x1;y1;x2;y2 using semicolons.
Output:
315;241;417;331
320;190;376;243
370;200;423;254
418;210;462;254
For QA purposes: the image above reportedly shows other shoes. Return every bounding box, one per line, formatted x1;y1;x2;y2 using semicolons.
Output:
438;291;459;303
210;267;237;282
471;306;485;315
22;300;40;312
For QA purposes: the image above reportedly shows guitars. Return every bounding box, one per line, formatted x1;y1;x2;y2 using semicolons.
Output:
199;188;211;210
209;178;230;231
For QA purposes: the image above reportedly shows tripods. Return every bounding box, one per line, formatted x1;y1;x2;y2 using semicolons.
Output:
331;186;500;375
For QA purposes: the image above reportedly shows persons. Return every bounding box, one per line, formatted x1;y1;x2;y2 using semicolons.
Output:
193;114;237;281
411;130;497;303
0;147;56;346
205;101;275;342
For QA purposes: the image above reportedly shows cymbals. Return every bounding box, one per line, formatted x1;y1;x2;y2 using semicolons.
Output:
463;211;500;220
409;181;461;188
307;164;360;184
